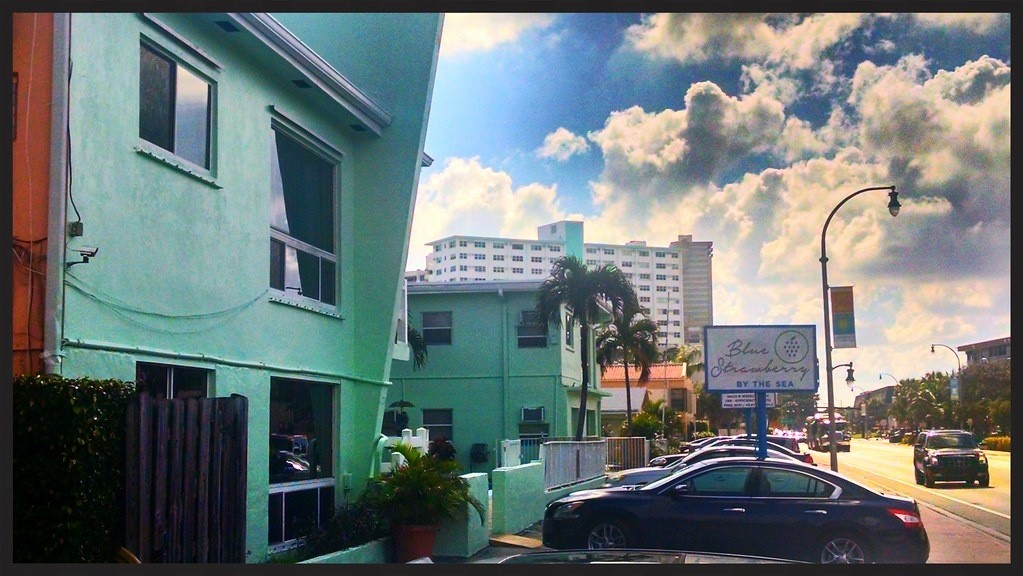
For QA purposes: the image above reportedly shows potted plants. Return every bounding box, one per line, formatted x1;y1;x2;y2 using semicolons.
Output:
357;438;487;563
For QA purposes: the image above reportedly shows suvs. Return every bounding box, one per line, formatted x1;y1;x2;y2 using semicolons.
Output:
912;429;989;488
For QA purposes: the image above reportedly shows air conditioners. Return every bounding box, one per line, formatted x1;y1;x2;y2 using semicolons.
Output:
521;406;545;422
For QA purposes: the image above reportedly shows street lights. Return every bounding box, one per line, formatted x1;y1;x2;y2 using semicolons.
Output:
826;361;856;473
878;372;905;420
819;184;904;475
931;343;964;429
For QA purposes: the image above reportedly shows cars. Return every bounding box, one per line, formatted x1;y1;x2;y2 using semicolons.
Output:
542;457;931;564
889;428;919;443
605;432;825;498
456;548;821;565
269;434;321;474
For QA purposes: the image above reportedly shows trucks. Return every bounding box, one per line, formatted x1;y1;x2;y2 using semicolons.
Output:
808;412;851;452
